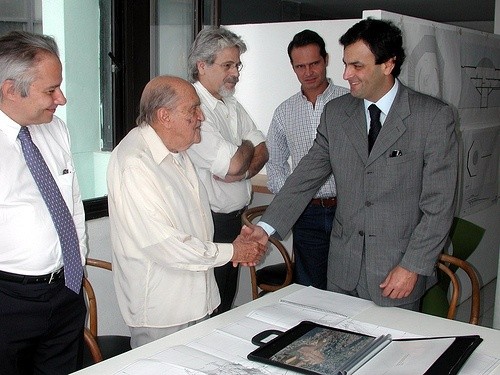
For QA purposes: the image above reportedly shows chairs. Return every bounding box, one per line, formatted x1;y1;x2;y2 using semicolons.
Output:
242;206;298;304
82;258;112;364
420;250;482;325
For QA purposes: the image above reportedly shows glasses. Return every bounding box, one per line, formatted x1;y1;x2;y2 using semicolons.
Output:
213;63;243;74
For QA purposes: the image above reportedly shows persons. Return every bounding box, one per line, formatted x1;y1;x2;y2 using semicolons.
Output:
264;29;351;292
232;16;463;315
187;26;269;319
106;75;269;350
1;32;89;375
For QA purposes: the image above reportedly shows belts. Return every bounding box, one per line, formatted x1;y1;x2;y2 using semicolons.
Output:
311;197;336;208
0;268;65;284
211;208;245;218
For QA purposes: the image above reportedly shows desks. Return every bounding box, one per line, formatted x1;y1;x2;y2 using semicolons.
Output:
67;283;500;375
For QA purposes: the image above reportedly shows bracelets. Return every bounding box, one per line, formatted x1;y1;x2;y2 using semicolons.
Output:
244;170;249;180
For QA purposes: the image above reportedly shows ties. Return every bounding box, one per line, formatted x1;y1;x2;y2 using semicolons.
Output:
17;126;85;294
368;104;382;157
